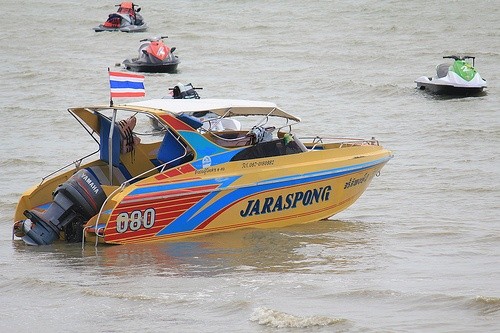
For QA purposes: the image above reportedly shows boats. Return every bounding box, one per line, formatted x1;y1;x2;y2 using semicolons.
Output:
12;99;393;251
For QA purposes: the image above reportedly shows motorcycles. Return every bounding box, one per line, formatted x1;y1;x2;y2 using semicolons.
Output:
415;56;488;96
116;37;181;72
92;2;148;32
168;82;203;99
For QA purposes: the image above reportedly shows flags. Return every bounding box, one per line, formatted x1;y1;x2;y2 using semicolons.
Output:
108;69;145;98
